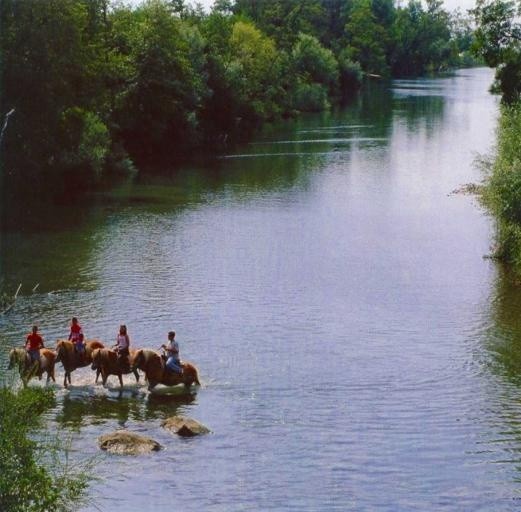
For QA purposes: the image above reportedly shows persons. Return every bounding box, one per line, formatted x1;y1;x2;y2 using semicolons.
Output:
24;325;46;370
162;330;185;376
113;324;130;367
68;317;84;363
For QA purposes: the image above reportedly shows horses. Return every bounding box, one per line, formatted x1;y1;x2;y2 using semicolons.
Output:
54;339;105;386
7;347;55;388
90;347;139;388
130;349;201;391
